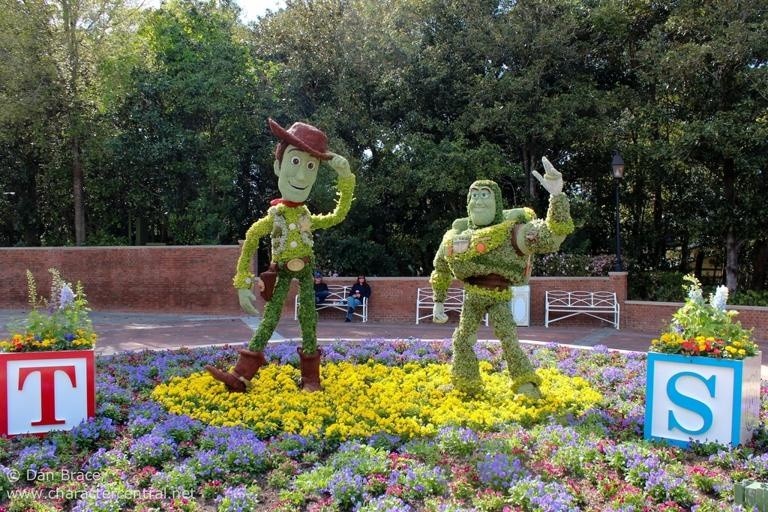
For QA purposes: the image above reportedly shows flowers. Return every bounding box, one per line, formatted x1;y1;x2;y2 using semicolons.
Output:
648;272;760;361
0;266;97;354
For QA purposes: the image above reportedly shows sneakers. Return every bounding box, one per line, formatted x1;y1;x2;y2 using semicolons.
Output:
344;307;355;322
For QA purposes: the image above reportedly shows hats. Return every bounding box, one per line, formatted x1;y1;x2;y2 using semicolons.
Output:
315;273;321;277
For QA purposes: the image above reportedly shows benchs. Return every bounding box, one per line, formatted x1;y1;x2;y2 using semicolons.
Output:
416;287;489;327
294;284;368;323
545;289;620;330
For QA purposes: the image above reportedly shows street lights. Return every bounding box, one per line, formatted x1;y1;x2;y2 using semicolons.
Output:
610;148;627;273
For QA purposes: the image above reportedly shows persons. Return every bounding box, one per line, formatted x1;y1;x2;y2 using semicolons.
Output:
344;273;371;323
313;271;330;308
